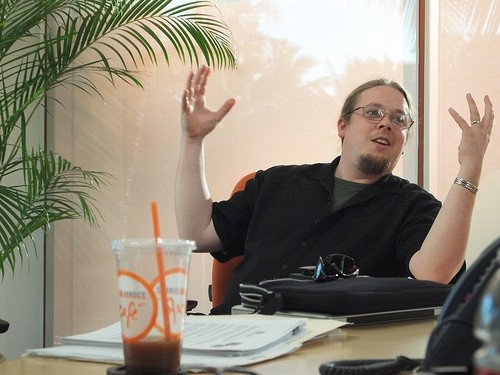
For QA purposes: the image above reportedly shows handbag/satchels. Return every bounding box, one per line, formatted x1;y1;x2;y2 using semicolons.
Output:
239;264;452;317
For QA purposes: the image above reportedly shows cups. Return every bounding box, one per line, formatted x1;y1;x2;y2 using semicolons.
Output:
111;238;197;375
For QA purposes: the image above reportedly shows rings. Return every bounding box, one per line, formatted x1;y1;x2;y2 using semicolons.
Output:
471;121;480;125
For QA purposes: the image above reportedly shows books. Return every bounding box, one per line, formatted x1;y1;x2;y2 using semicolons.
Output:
232;304;441;327
56;313;308;357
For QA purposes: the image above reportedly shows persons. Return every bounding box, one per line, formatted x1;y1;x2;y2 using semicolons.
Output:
175;65;495;315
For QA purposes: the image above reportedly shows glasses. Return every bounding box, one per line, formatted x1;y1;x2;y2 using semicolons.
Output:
347;104;414;130
312;254;359;283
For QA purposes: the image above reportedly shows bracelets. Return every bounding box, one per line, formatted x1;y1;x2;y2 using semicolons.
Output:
454;177;479;195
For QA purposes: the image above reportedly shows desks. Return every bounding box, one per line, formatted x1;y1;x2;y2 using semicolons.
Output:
1;321;437;375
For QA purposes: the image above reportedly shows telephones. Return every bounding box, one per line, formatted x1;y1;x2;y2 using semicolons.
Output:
320;234;500;375
